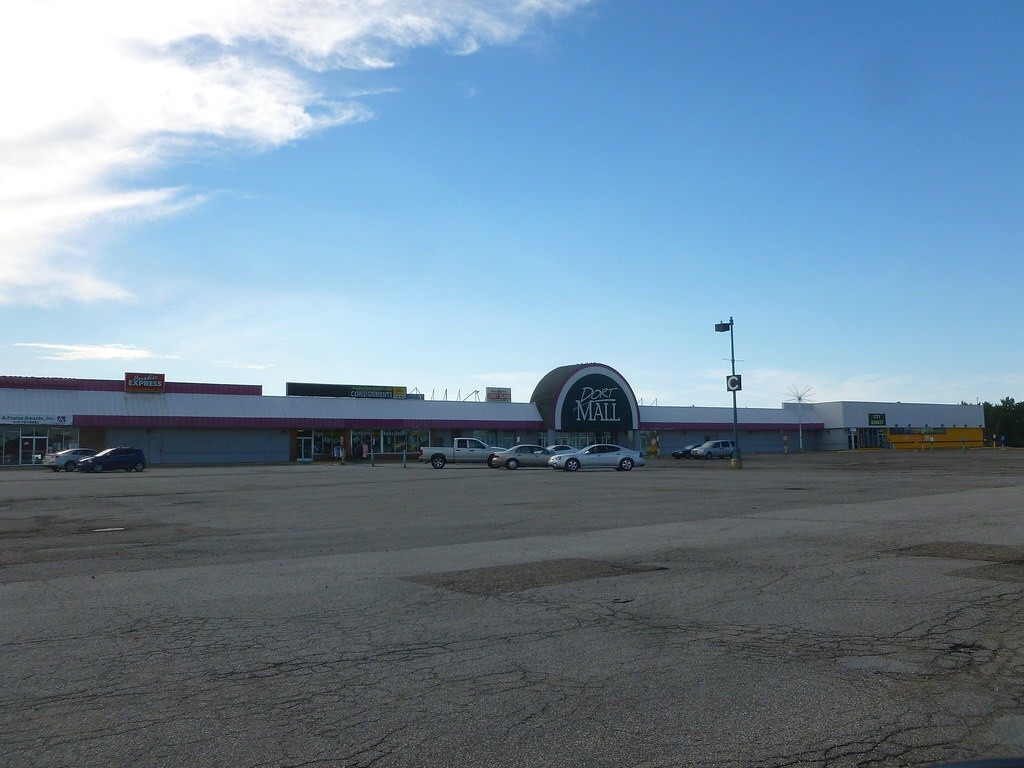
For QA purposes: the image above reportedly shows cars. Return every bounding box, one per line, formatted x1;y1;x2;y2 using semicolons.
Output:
671;444;703;460
490;443;558;470
533;444;579;455
75;446;147;474
547;443;648;471
41;447;99;472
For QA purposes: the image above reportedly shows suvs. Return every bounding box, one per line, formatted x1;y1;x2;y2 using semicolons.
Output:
690;439;740;461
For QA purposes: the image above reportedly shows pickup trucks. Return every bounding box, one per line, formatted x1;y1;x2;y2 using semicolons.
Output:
418;437;509;470
